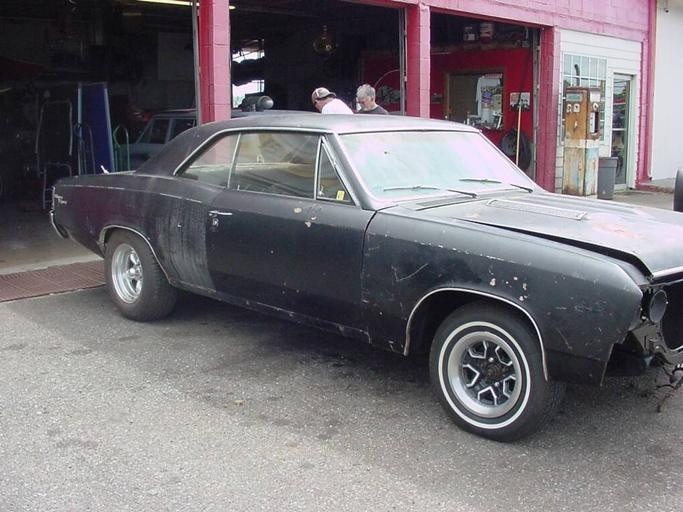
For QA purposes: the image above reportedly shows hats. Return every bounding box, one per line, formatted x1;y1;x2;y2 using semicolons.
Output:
311;87;337;105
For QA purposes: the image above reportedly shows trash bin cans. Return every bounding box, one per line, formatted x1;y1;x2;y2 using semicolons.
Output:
598;157;619;200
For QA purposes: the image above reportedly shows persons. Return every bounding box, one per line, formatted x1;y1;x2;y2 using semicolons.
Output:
356;84;389;115
310;87;353;114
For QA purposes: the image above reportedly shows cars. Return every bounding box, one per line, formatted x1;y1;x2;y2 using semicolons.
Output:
50;111;683;443
120;94;324;170
